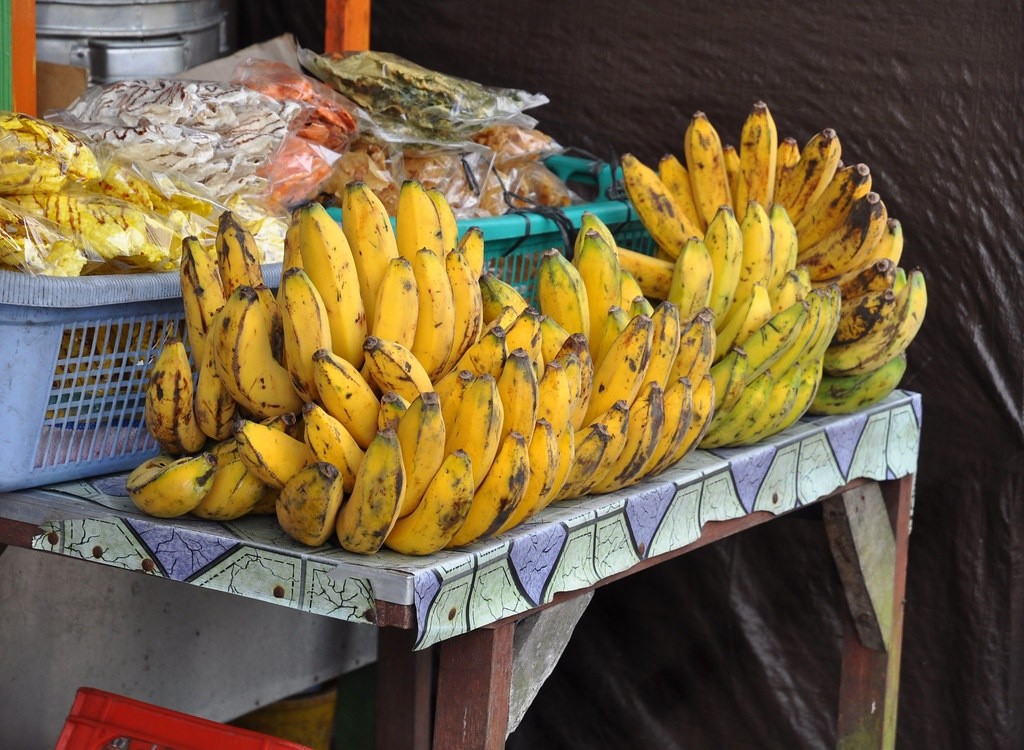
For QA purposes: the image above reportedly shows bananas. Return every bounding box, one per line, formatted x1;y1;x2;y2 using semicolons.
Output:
125;96;928;556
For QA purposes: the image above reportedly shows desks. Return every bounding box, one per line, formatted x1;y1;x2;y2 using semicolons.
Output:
1;383;924;750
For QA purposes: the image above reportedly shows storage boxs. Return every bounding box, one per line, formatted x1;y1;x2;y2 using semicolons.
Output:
54;686;311;750
0;154;661;495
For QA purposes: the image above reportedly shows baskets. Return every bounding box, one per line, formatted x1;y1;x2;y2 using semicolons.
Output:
0;264;285;492
322;152;660;318
